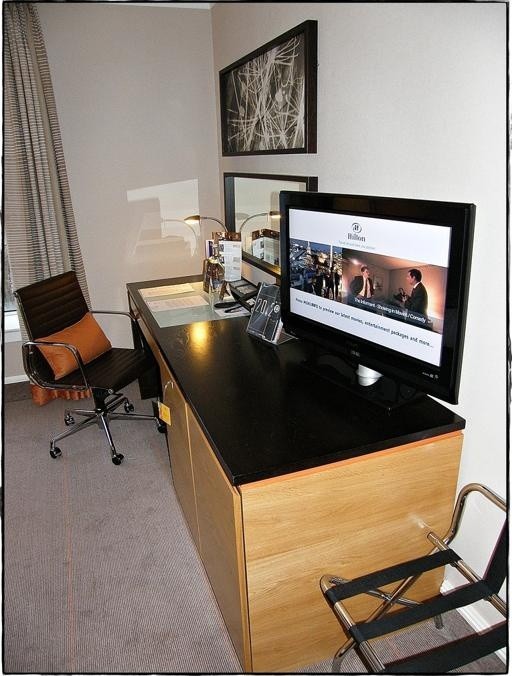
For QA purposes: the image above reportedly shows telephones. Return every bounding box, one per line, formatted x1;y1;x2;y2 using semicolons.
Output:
238;289;259;312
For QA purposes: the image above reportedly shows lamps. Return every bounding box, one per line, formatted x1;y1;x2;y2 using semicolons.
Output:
183;215;241;240
239;211;280;234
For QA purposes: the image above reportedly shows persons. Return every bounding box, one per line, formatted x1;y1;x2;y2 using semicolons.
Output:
350;266;375;312
402;269;428;330
394;288;408;303
303;270;340;299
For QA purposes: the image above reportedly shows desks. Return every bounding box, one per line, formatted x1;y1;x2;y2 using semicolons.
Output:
126;272;467;675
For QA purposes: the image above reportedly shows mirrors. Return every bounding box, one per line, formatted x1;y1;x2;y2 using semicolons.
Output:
224;171;318;280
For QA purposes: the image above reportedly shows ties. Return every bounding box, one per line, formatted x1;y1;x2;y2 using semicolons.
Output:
364;279;367;298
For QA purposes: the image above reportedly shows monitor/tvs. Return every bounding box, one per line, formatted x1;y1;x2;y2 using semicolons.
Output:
279;190;475;410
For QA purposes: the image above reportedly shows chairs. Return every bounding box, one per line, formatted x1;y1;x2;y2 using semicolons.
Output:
316;482;507;675
13;270;166;465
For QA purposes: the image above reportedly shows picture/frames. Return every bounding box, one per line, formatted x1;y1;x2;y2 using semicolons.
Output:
218;19;317;156
